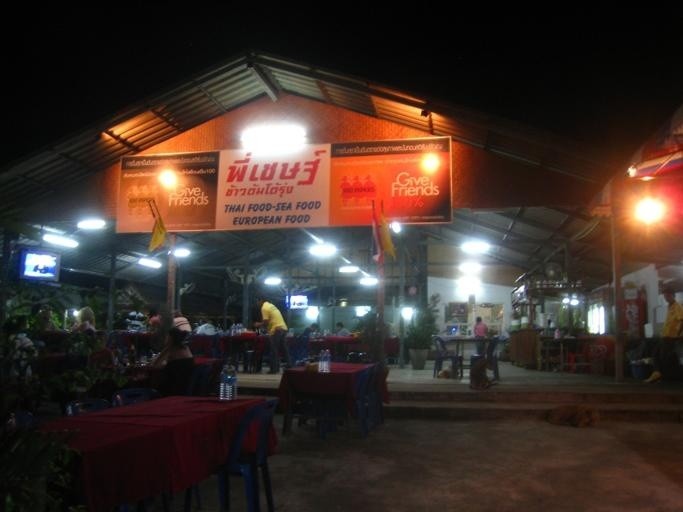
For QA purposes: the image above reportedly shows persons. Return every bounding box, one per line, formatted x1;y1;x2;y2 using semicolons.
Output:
301;322;318;348
642;287;682;383
473;317;488;353
3;294;254;398
252;294;292;376
334;321;349;337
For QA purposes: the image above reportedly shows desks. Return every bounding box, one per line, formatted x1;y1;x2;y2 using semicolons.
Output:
449;338;506;381
192;333;358;372
543;338;594;374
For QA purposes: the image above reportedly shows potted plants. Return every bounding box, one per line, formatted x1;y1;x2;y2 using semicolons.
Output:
405;293;439;369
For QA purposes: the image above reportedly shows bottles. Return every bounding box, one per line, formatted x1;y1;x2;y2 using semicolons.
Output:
318;349;331;371
220;365;238;400
555;328;560;338
232;323;242;334
452;328;456;334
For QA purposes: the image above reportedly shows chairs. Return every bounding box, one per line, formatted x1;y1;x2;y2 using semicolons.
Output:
471;335;499;380
186;397;278;509
432;335;463;380
357;363;380;437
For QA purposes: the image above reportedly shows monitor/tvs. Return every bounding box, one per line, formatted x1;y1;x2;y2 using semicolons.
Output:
285;295;309;310
19;249;61;282
